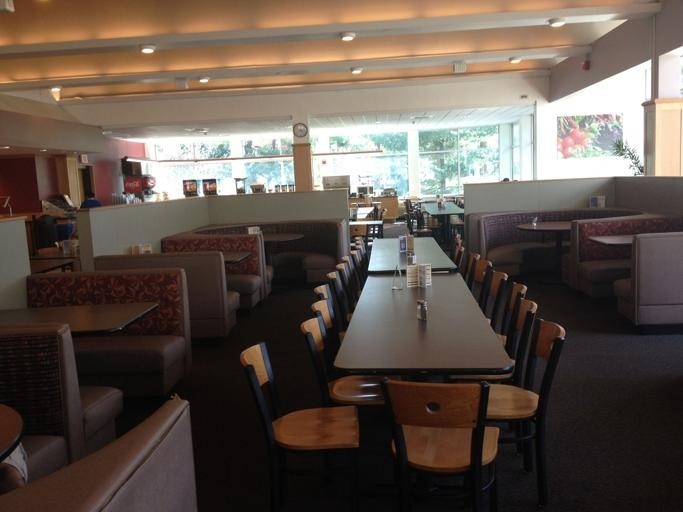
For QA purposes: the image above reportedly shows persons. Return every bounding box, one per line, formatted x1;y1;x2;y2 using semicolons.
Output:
78;191;101;209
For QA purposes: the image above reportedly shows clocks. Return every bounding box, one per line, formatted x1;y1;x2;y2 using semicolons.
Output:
293;123;308;137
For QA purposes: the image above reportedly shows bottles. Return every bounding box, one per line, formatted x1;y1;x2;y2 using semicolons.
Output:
120;242;152;256
407;252;415;265
437;198;449;210
111;191;141;205
415;300;427;322
70;241;80;255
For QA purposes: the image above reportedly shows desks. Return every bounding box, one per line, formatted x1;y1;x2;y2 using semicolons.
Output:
350;221;384;248
588;235;648;276
517;220;571;286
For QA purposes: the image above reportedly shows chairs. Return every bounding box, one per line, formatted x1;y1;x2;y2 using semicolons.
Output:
375;209;387;221
405;196;464;230
239;229;567;512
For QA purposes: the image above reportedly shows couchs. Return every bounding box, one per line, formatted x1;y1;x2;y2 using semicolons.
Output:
194;220;346;283
466;208;670;297
613;232;683;326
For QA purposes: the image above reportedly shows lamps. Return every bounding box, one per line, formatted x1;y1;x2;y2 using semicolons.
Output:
51;86;62;91
350;67;364;73
510;57;521;63
139;44;155;53
340;31;355;40
198;76;210;82
549;18;566;26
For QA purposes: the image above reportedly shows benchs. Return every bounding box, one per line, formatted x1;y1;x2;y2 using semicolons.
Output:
0;232;273;512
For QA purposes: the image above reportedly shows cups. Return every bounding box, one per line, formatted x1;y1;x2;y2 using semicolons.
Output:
281;185;286;192
287;185;294;192
62;240;72;256
528;216;536;226
267;186;273;192
274;185;280;192
